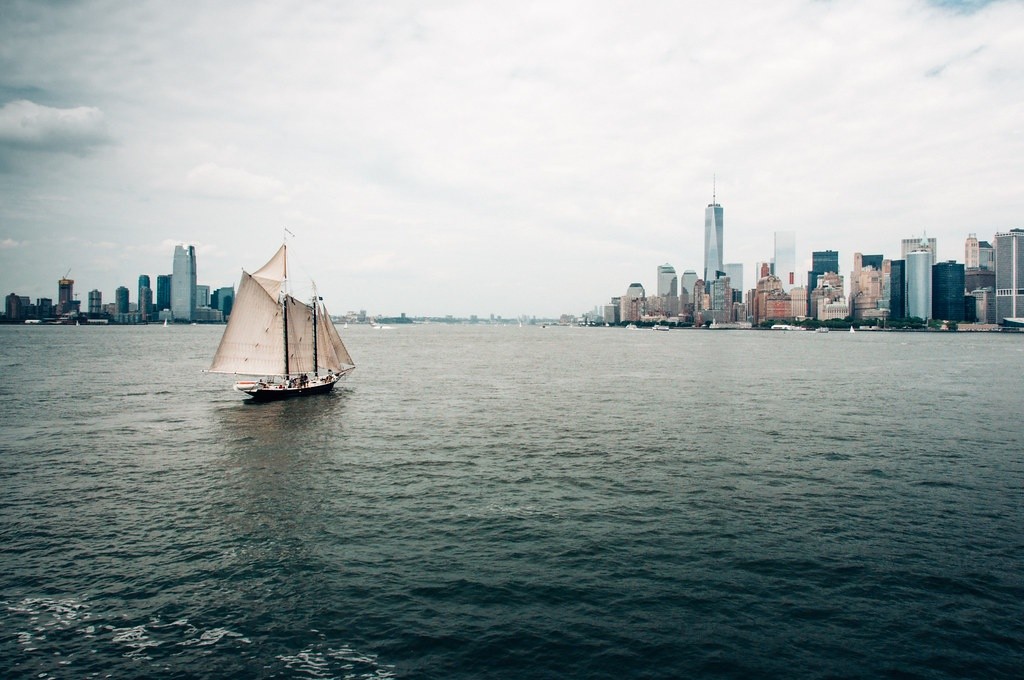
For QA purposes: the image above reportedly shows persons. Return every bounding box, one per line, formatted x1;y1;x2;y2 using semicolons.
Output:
260;372;334;388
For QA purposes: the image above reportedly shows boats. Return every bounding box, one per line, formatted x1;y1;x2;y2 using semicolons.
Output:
815;326;830;333
626;323;641;330
652;324;670;331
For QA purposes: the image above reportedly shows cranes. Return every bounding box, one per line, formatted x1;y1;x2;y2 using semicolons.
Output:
62;267;72;280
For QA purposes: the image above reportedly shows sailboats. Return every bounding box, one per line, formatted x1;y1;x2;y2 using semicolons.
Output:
201;227;358;399
